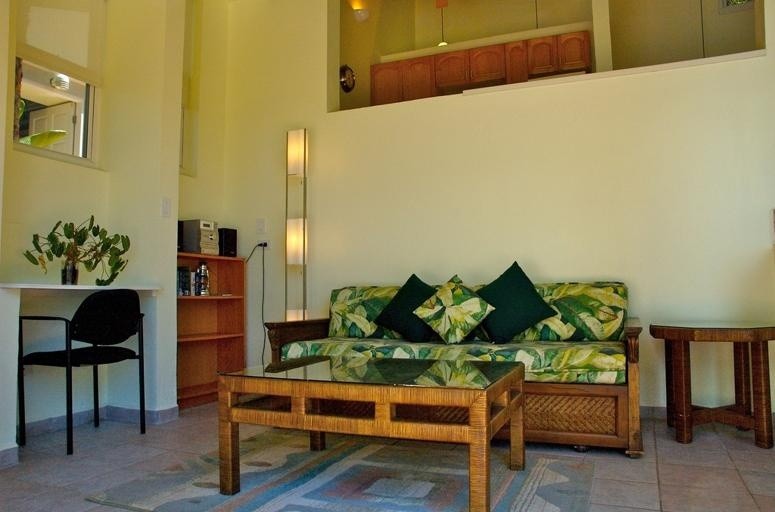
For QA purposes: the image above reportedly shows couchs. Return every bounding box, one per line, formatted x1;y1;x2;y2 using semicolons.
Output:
263;281;646;459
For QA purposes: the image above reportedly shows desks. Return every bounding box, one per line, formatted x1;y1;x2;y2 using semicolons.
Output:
0;284;159;470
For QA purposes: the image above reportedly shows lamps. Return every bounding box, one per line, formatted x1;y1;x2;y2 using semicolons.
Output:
285;128;307;323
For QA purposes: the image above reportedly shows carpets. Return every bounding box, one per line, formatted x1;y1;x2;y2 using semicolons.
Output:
83;425;597;511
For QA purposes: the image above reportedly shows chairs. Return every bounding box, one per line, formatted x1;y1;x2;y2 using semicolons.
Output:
17;288;145;454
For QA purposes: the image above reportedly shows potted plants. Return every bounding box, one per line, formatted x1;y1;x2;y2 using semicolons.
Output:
22;214;129;286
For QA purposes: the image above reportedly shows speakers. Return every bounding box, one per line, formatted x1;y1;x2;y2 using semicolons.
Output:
178;222;184;252
219;228;237;257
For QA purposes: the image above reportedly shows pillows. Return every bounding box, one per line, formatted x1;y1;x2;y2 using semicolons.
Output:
374;259;559;344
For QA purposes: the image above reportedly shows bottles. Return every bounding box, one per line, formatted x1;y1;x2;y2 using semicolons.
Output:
195;258;211;296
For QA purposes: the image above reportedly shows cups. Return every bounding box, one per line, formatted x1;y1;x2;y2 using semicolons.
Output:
62;259;78;284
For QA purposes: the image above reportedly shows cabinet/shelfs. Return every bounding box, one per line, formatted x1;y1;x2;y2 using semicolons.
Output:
176;254;248;409
370;21;593;108
